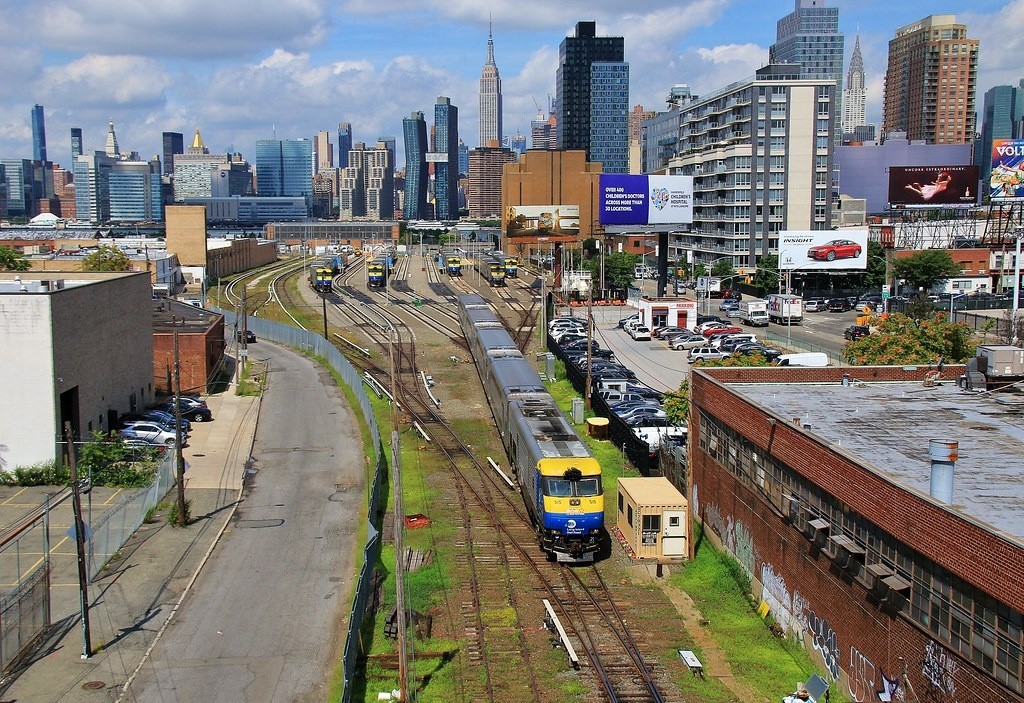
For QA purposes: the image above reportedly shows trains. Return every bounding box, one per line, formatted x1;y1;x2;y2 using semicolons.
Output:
438;246;462;277
465;247;518;286
310;250;349;294
368;245;399;288
457;293;606;564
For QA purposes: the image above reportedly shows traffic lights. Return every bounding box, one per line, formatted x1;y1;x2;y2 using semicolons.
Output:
744;270;745;275
738;269;742;276
748;277;751;284
745;277;749;285
741;270;743;275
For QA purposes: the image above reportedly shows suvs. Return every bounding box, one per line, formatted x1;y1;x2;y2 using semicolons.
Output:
719;298;738;310
686;346;732;365
829;298;852;313
843;324;871;341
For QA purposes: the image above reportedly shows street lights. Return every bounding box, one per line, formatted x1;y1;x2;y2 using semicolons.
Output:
873;255;888;315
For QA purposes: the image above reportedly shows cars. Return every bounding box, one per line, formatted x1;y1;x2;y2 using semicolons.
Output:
728;306;742;318
115;396;211;462
547;314;685;474
893;289;1024;303
672;335;712;351
694;312;784;362
659;327;694;341
806;239;862;260
805;300;828;312
668;334;691;347
848;291;883;312
633;263;693;295
234;330;257;343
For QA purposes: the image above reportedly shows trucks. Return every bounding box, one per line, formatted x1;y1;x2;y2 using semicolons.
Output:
764;293;804;326
738;299;770;326
694;276;721;298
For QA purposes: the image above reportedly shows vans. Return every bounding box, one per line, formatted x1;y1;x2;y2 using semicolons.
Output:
771;352;829;368
